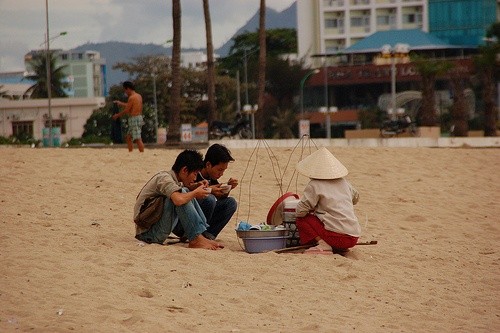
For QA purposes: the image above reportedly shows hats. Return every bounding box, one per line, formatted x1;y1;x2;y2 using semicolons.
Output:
295;146;348;179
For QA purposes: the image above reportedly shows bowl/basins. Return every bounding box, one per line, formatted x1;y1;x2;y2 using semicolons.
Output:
204;188;212;195
219;185;232;193
240;235;292;252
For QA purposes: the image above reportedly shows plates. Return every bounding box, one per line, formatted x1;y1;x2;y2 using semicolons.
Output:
235;227;286;237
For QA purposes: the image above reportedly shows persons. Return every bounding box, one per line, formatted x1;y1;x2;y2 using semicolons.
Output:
112;81;145;152
171;144;238;243
133;148;224;250
294;146;361;252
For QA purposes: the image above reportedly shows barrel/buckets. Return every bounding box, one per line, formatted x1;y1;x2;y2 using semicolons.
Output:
282;200;302;248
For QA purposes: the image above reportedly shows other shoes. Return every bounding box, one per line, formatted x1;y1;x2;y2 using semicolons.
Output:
302;240;319;249
332;248;348;252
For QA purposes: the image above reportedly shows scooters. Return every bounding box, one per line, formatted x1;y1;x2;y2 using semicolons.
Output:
380;111;419;138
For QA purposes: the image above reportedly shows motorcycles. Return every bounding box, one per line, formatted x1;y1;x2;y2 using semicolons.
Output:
207;112;253;141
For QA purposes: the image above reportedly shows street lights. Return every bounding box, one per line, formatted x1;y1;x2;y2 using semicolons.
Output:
152;39;174;142
41;31;68;147
381;42;411;122
300;69;320;121
